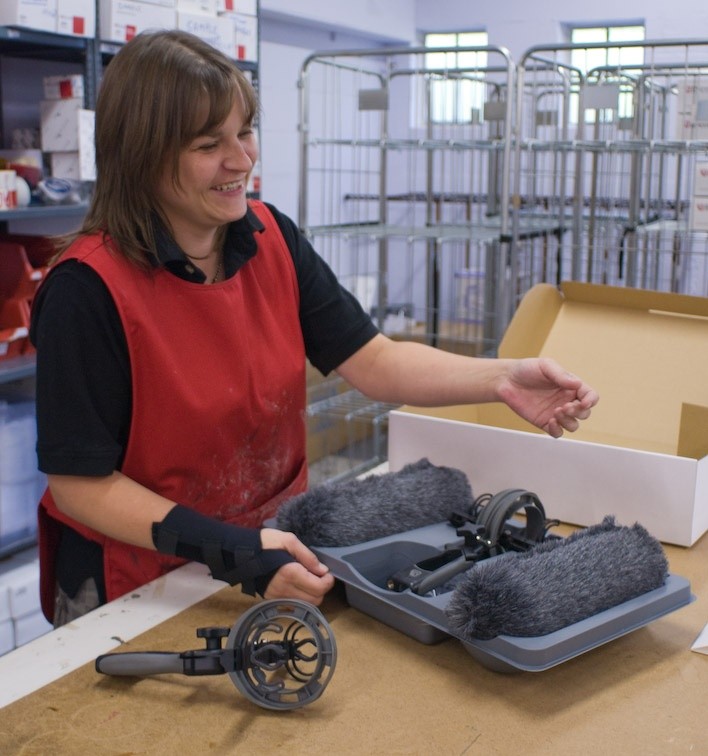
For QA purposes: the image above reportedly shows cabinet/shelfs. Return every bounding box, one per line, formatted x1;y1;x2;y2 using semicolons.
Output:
0;26;265;555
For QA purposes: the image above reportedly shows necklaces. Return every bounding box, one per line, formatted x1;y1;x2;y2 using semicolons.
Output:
183;247;219;262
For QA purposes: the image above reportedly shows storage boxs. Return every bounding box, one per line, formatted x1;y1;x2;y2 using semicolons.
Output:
0;0;259;209
387;278;708;550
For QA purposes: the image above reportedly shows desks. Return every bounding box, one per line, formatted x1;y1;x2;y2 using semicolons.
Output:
0;457;708;756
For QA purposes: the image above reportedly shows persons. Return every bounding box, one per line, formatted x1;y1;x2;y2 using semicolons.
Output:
30;28;598;629
